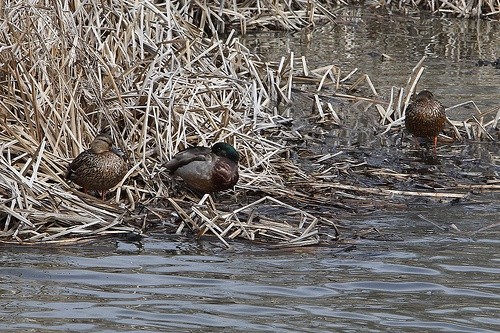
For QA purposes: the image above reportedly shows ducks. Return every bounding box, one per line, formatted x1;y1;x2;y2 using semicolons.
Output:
405;91;446;154
163;142;240;202
70;132;128;201
